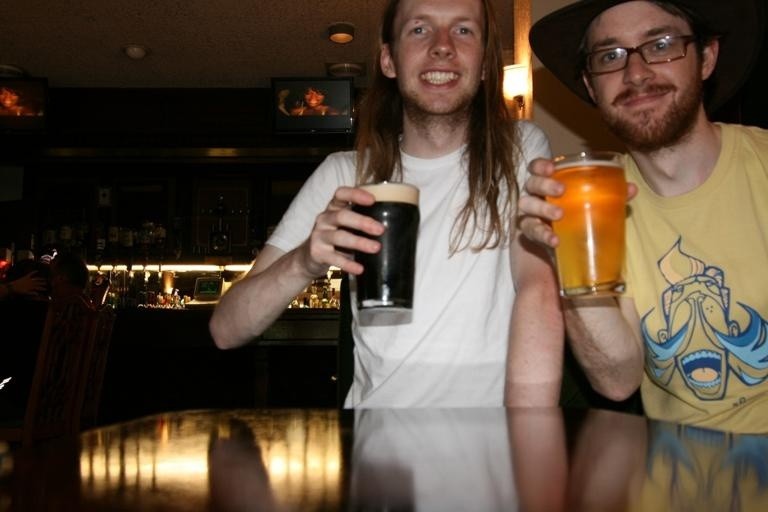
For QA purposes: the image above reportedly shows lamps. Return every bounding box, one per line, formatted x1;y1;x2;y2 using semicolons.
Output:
122;43;151;61
327;22;355;44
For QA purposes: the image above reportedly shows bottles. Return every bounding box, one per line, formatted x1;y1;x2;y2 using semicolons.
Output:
86;269;168;312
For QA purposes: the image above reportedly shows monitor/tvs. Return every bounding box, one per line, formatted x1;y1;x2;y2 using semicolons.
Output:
0;76;48;126
271;75;355;136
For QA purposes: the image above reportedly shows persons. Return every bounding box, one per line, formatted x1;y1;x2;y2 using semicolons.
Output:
208;0;568;409
513;1;768;436
0;267;54;417
564;410;768;512
198;409;564;511
283;78;338;116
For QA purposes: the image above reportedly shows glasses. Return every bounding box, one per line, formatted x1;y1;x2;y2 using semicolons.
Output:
579;29;707;77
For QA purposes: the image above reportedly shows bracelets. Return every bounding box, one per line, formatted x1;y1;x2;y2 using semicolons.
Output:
7;281;16;295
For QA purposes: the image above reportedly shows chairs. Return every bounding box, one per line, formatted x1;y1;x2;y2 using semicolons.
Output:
72;305;118;420
0;294;96;448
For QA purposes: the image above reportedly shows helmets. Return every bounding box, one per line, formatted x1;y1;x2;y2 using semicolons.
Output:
529;1;764;115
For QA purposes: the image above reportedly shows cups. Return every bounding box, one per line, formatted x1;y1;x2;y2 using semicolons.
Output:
350;181;420;316
542;150;626;300
